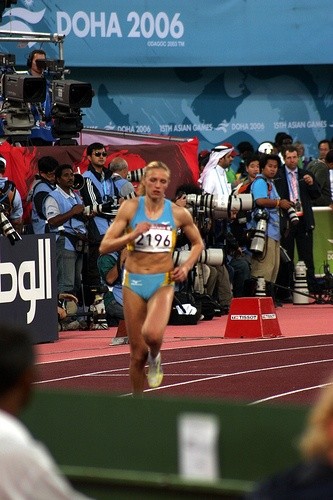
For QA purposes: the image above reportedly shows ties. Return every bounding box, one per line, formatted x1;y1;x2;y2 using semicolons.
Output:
290;170;298;203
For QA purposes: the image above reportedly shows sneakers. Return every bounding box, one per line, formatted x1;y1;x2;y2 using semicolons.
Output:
147;352;163;388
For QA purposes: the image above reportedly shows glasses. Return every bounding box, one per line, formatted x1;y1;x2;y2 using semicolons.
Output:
91;152;107;157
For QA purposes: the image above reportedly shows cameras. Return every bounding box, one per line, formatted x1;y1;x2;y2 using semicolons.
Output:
172;191;270;267
254;276;267;296
0;204;23;246
72;201;113;219
283;201;299;225
126;167;146;182
291;260;309;305
70;174;84;192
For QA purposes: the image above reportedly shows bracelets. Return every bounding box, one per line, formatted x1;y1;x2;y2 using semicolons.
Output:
277;200;280;207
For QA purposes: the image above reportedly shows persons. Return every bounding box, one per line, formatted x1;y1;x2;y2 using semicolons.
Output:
240;375;333;500
170;183;232;317
99;160;204;398
0;95;9;136
0;321;94;500
20;50;60;144
197;132;333;303
0;142;139;329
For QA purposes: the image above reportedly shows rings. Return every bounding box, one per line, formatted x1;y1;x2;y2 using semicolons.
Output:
286;208;287;210
